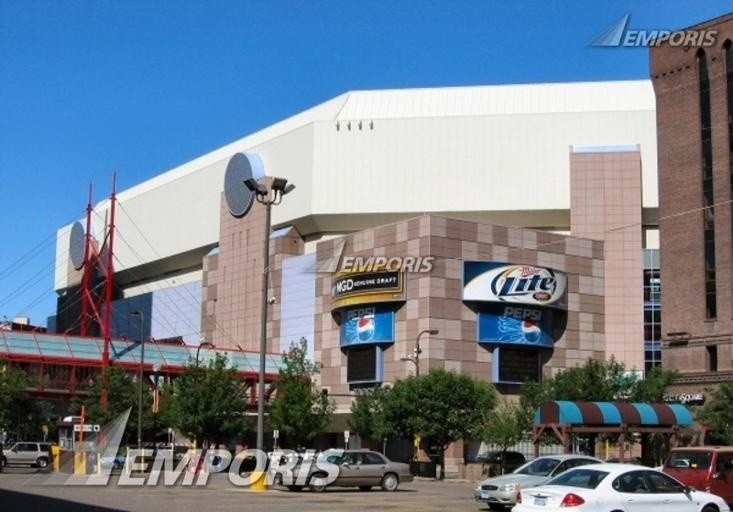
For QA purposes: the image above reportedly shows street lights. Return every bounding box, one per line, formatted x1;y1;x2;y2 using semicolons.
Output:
244;178;296;447
131;310;144;448
195;341;212;364
400;330;439;376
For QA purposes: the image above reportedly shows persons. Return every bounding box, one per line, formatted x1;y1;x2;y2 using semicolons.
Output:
41;422;50;442
488;456;506;476
0;426;7;448
96;431;109;450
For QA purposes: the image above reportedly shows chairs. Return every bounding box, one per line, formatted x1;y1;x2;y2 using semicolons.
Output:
615;476;646;492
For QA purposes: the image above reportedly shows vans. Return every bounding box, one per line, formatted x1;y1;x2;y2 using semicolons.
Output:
3;443;56;468
661;446;732;506
476;451;524;468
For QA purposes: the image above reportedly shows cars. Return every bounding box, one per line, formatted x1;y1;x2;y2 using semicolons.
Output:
102;452;126;469
512;461;730;512
473;454;604;510
275;449;412;491
